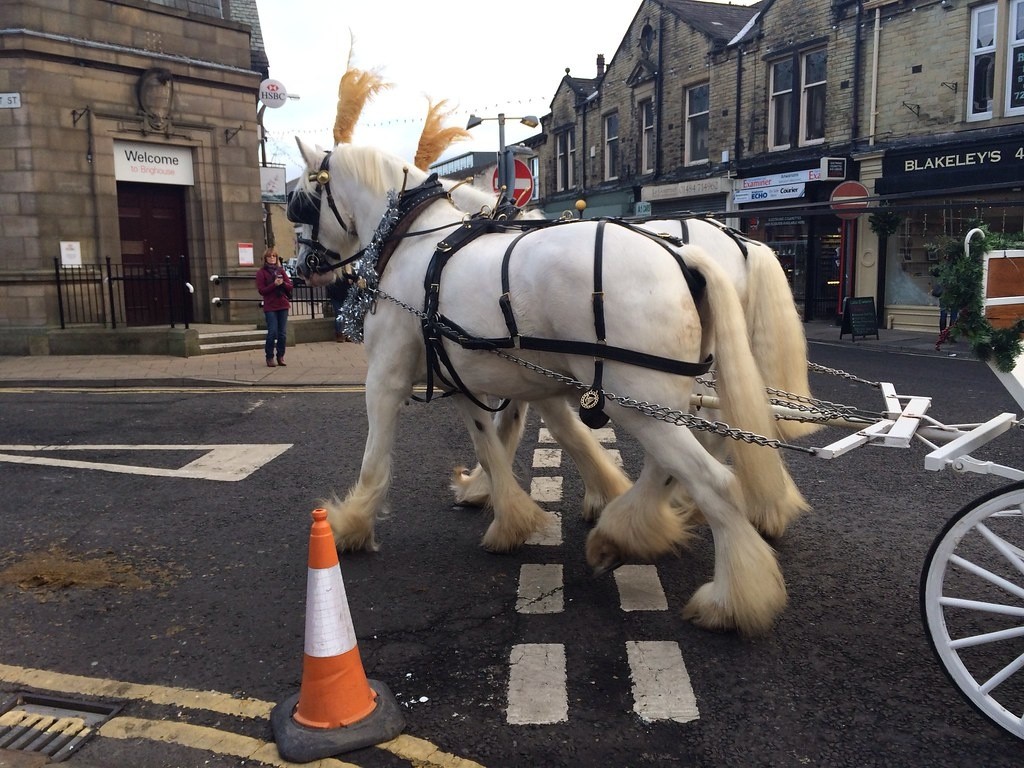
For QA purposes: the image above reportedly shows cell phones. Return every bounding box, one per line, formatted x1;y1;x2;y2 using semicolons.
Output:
277;275;283;278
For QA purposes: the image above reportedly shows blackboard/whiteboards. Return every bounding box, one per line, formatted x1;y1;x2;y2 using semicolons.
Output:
840;296;878;335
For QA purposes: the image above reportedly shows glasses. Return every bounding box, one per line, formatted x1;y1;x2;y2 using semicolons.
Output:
266;254;276;257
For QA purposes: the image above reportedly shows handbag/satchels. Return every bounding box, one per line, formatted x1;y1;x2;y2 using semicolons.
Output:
279;267;292;299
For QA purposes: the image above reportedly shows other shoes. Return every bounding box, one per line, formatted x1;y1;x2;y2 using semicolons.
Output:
277;356;286;366
266;358;275;367
335;332;344;342
346;335;353;342
947;337;958;344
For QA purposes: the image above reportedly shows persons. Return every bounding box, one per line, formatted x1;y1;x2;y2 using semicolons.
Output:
325;271;357;342
932;267;958;344
255;248;292;366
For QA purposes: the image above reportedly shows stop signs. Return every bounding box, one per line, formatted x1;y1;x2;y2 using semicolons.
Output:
492;159;534;208
830;180;869;221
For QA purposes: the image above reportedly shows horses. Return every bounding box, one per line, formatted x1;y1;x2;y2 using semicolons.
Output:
276;134;826;637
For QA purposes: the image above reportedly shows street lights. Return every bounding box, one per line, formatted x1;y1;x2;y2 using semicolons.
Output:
466;111;538;197
574;199;586;219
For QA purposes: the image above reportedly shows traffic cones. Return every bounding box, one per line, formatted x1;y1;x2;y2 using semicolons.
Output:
269;508;407;764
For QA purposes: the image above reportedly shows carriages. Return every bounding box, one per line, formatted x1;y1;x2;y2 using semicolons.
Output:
286;64;1024;747
